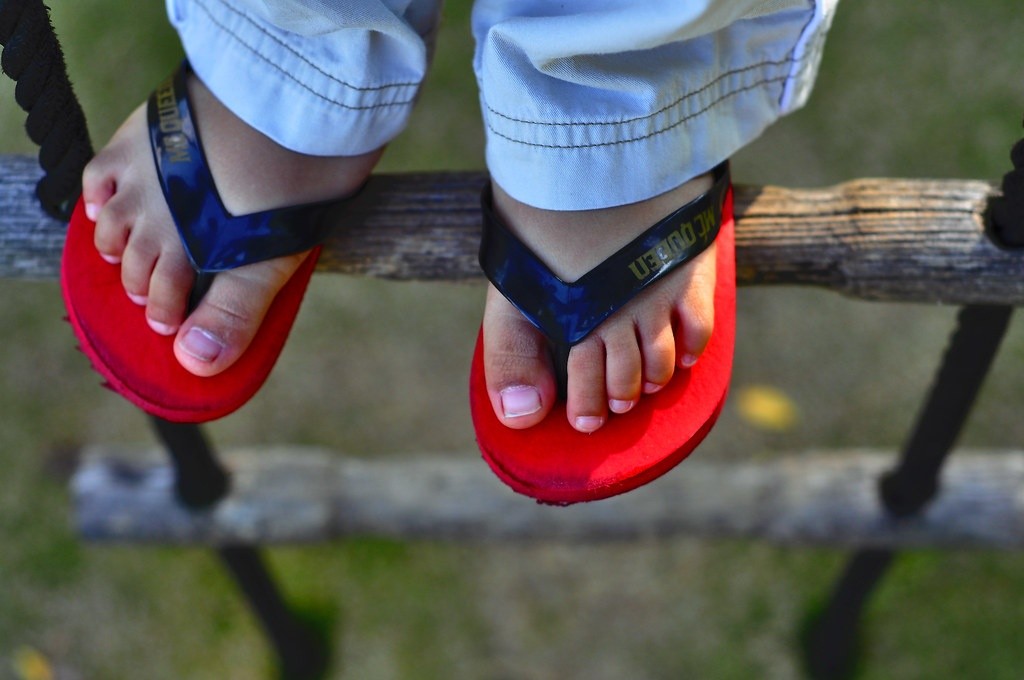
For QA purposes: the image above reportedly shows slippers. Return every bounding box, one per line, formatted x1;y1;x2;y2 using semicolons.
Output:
471;159;737;507
60;57;372;424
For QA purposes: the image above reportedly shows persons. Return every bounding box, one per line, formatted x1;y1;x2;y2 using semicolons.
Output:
60;0;837;508
